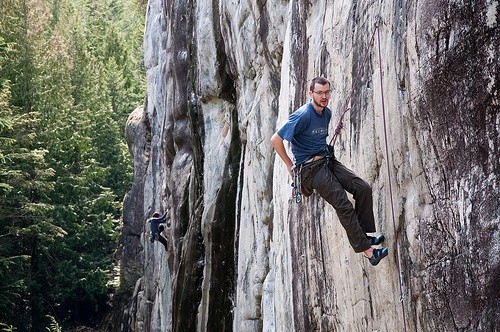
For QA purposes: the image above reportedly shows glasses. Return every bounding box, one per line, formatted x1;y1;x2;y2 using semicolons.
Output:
313;90;331;94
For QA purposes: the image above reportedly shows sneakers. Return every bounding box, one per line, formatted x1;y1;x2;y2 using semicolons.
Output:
369;248;388;266
365;235;385;245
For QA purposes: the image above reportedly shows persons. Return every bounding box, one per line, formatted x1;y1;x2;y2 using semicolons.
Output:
146;210;174;252
270;75;390;268
